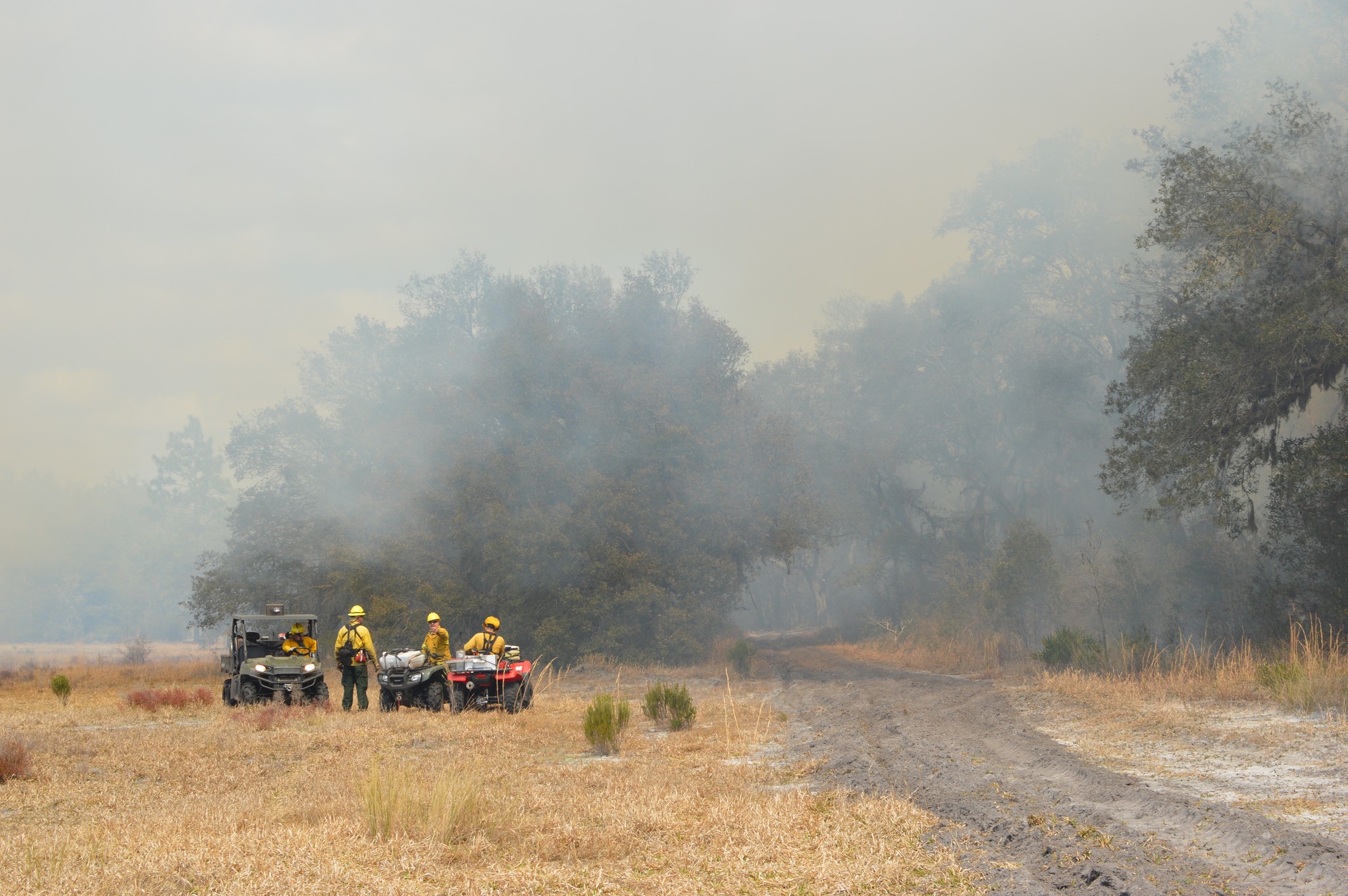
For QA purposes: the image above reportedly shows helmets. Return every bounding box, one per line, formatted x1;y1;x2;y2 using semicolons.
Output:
348;605;366;616
427;612;441;622
289;624;304;634
482;616;500;630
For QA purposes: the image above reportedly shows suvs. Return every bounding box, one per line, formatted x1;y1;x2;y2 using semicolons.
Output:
220;604;329;708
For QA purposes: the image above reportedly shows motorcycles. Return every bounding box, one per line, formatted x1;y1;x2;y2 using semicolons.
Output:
445;631;534;715
377;647;448;713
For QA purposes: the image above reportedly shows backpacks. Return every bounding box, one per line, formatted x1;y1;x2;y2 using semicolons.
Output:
339;641;354;666
354;648;367;662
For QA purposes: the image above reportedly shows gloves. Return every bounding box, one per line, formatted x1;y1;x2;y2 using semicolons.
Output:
309;652;315;658
290;647;298;651
336;663;343;672
374;661;379;672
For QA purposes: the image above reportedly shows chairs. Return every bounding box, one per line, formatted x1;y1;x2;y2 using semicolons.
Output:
239;632;265;669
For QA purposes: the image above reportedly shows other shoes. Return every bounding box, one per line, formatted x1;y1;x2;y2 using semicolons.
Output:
343;707;350;712
358;706;367;711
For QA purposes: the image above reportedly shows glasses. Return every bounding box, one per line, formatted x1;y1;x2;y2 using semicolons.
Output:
361;616;364;618
429;620;438;624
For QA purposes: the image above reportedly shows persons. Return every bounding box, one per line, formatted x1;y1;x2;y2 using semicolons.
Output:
462;616;505;660
423;613;452;675
283;623;317;659
335;605;379;713
